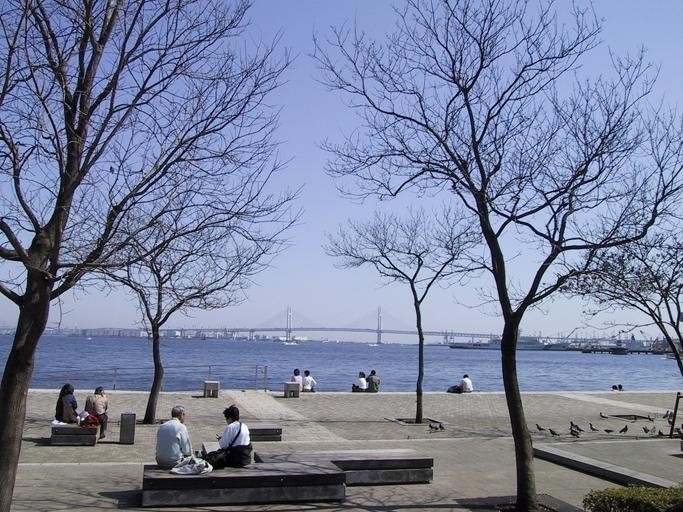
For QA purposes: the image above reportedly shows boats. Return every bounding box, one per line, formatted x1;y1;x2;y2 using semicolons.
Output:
282;341;300;346
368;343;379;347
447;329;683;360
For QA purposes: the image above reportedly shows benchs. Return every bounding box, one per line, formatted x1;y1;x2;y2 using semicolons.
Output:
142;462;346;508
254;449;433;484
51;422;101;446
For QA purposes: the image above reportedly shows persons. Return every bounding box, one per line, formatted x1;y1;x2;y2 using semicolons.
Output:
456;374;474;393
302;370;318;392
352;371;367;392
290;368;303;392
618;384;625;391
54;382;79;424
215;404;253;468
154;405;194;468
611;385;619;392
84;385;109;439
365;369;381;393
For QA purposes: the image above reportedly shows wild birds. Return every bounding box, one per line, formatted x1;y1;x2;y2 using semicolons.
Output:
569;421;584;438
599;412;609;420
647;415;653;422
589;422;599;431
536;423;545;433
658;430;663;436
428;423;445;433
642;425;657;433
604;429;614;434
619;425;628;434
662;409;682;438
548;429;558;437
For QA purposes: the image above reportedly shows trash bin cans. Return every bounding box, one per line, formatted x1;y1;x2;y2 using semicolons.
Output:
120;414;136;444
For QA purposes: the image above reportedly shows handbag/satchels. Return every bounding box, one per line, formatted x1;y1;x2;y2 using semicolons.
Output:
205;448;230;469
80;415;99;426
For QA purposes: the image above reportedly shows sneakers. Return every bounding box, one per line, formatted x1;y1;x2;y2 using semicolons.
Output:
100;432;105;438
